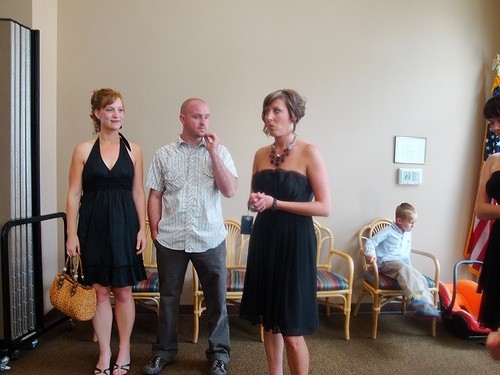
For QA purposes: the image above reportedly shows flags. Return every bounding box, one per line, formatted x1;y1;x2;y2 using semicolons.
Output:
464;81;500;277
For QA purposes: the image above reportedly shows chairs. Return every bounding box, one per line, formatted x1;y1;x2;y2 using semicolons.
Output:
91;219;161;343
192;219;265;345
357;218;441;339
311;219;352;340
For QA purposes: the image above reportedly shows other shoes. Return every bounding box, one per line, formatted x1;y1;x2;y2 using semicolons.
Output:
415;303;440;318
410;294;428;307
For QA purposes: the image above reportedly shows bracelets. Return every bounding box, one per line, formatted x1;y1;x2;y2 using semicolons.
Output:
272;199;277;209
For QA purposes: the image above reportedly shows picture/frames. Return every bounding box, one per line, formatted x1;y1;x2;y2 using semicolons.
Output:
394;135;428;166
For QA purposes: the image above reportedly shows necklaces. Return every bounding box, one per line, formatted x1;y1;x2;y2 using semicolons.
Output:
269;136;296;166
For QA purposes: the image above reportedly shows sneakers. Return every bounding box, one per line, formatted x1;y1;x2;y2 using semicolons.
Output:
210;359;227;375
142;355;166;375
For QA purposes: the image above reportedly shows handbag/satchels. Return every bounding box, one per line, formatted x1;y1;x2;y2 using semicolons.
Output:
49;252;97;321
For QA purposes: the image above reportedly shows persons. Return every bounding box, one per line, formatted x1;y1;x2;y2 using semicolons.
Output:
146;98;239;375
239;89;330;374
65;88;147;375
474;86;500;331
364;203;438;316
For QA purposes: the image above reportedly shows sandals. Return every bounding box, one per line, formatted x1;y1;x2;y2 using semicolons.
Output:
93;355;111;375
113;352;132;375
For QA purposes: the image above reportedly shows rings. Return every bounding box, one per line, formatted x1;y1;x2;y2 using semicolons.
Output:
69;251;71;253
